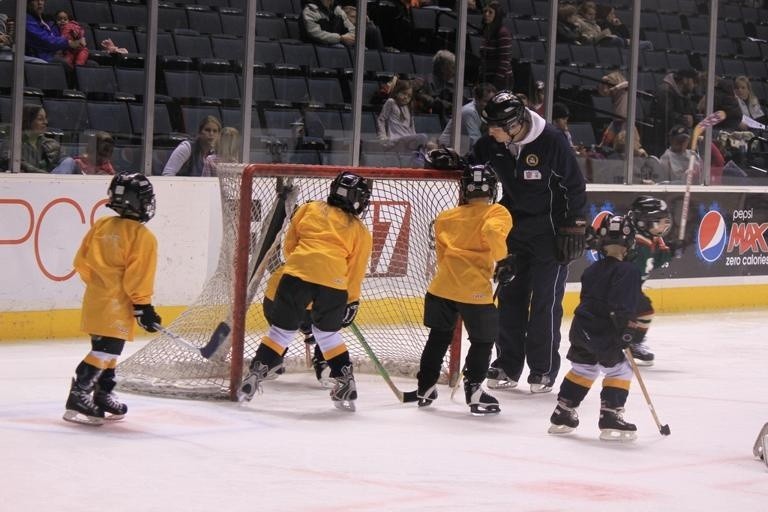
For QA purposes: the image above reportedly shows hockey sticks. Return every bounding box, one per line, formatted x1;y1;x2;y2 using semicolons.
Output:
151;321;231;360
450;282;502;400
608;309;673;436
349;320;418;402
675;110;728;259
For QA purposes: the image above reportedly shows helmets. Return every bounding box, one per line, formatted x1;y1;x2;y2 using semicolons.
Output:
631;195;673;238
78;130;114;165
598;214;635;247
460;164;498;204
327;171;370;214
106;170;156;221
481;90;525;129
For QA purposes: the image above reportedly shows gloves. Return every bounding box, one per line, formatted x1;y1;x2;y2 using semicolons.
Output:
341;301;359;328
494;254;518;285
556;221;586;261
133;304;161;332
613;320;638;350
425;148;459;168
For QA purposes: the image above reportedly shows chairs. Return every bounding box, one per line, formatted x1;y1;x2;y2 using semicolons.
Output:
0;0;768;184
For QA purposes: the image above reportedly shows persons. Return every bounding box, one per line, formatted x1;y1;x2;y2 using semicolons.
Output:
549;215;641;433
416;163;514;409
66;170;158;420
202;125;240;176
160;114;221;176
456;91;588;387
240;170;373;402
74;132;117;174
0;0;100;98
301;2;583;169
1;103;77;175
624;196;692;360
558;2;767;184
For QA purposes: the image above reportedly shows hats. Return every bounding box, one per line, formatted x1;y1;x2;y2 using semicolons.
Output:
552;102;569;120
669;124;690;140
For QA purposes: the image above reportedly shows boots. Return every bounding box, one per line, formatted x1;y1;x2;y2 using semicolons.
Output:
311;356;357;401
487;367;506;379
599;398;636;430
463;377;498;406
66;377;104;417
528;373;555;387
418;384;437;400
551;397;579;427
94;380;127;414
629;335;654;360
242;354;284;395
